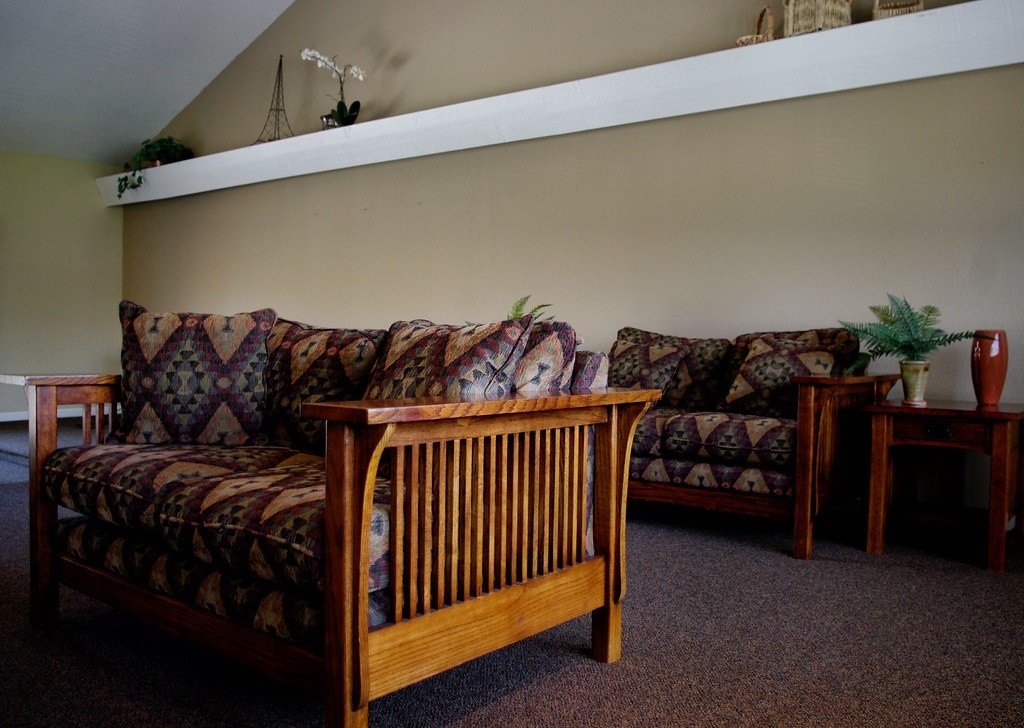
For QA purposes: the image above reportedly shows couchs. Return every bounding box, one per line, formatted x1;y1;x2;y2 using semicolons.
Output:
26;299;662;728
606;328;902;560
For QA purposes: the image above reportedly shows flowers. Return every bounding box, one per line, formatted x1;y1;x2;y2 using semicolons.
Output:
299;47;367;102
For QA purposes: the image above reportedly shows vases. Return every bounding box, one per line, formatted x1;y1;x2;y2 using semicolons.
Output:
320;101;361;131
970;329;1008;405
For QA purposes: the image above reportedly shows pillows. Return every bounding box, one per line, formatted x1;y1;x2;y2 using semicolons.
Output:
723;335;837;419
512;321;574;404
605;338;691;408
105;301;277;449
261;318;375;453
361;313;533;404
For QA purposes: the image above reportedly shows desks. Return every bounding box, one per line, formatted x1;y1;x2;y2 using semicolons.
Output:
867;399;1024;573
0;373;117;472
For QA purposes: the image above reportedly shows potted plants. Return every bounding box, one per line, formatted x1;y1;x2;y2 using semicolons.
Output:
834;291;1002;406
115;135;196;200
